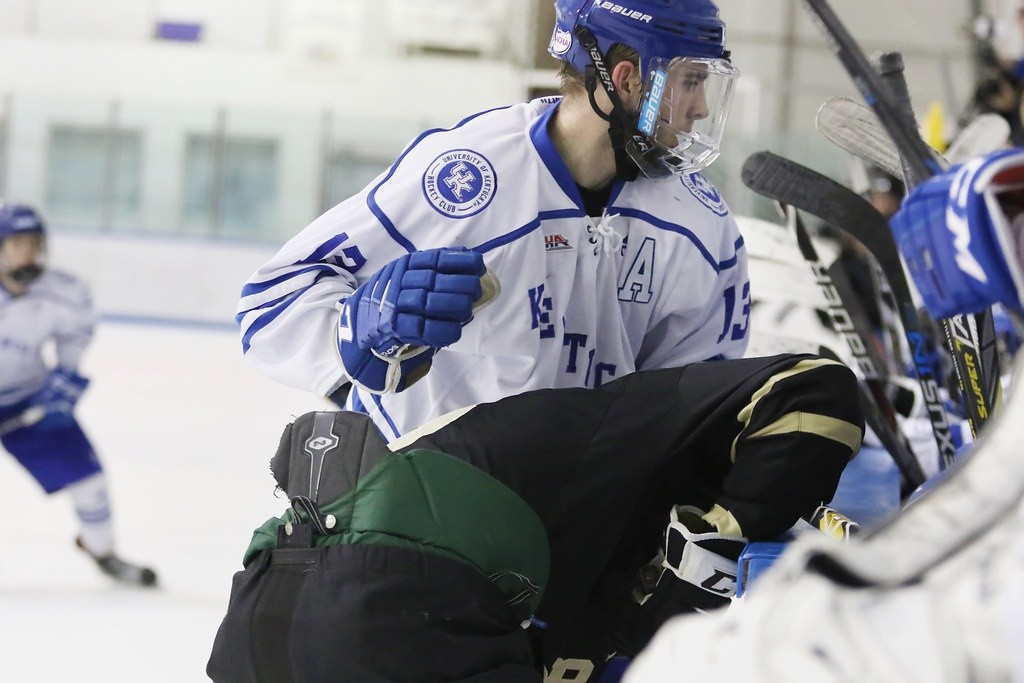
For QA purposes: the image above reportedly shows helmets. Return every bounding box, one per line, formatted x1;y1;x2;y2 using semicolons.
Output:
547;0;726;94
0;204;44;246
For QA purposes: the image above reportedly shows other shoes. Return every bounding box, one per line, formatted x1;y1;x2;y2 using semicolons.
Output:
76;537;155;590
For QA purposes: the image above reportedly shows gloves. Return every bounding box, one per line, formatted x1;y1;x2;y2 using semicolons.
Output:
637;506;749;647
335;246;499;395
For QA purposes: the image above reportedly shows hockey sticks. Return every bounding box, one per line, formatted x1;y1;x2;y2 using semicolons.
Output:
804;0;1004;443
776;197;929;489
739;146;957;472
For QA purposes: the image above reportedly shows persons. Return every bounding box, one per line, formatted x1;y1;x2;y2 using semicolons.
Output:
206;351;863;683
0;203;156;586
232;0;753;445
622;55;1024;682
